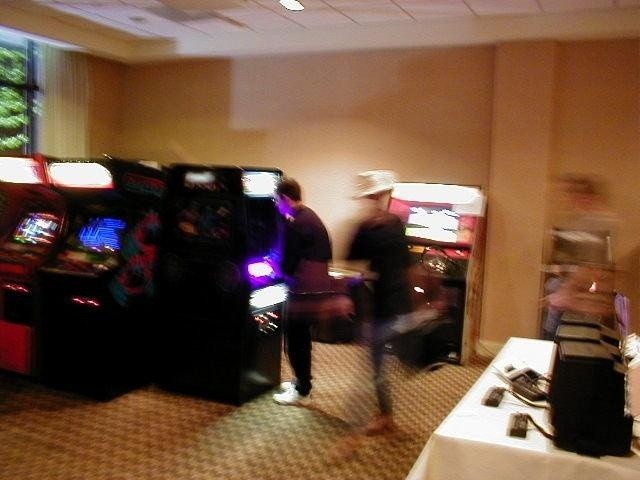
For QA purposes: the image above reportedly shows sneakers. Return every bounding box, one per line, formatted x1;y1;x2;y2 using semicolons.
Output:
272;382;311;407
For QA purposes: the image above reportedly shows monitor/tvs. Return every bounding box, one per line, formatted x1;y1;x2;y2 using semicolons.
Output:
8;209;66;248
547;312;633;458
385;183;482;248
73;217;127;251
242;252;283;289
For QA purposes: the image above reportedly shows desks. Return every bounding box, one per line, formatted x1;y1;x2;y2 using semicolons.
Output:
399;332;640;480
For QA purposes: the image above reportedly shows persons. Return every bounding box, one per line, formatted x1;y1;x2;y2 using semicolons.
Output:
272;178;331;406
333;170;415;437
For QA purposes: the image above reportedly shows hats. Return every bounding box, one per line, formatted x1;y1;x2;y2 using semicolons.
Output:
347;169;397;200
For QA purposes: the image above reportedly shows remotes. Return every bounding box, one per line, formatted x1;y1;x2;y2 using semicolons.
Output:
485;388;505;407
511;414;528;437
